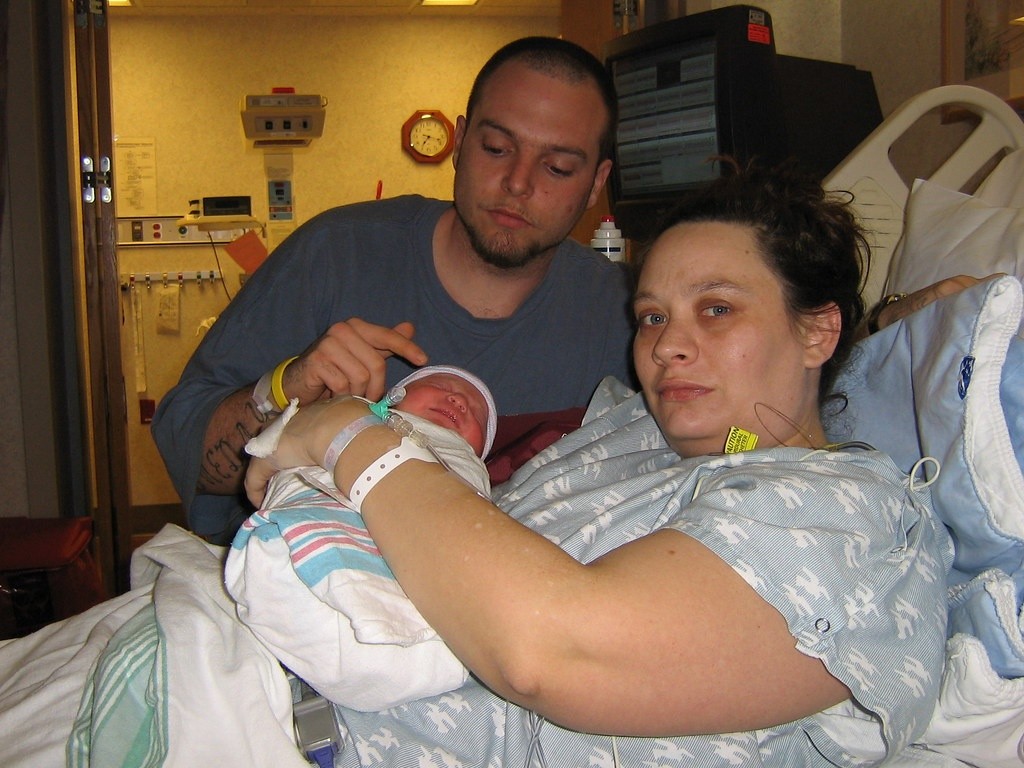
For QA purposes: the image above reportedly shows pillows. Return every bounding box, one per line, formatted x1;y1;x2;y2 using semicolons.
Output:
823;180;1024;744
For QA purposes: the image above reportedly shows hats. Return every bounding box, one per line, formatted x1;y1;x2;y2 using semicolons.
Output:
395;365;497;462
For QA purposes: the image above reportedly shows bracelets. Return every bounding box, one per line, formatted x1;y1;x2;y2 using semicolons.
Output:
253;371;281;414
868;292;908;332
349;437;439;512
324;414;385;475
271;355;299;414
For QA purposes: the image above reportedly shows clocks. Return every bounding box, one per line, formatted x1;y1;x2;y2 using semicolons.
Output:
402;110;455;164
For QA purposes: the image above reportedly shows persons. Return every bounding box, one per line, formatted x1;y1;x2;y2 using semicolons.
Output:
152;37;1003;533
226;362;497;712
1;171;957;768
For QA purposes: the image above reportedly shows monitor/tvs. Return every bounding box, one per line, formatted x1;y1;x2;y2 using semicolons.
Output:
601;5;885;245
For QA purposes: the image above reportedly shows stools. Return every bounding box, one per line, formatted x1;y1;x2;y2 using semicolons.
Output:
0;513;109;637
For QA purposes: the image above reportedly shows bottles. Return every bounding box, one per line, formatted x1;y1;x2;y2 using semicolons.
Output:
590;215;626;263
